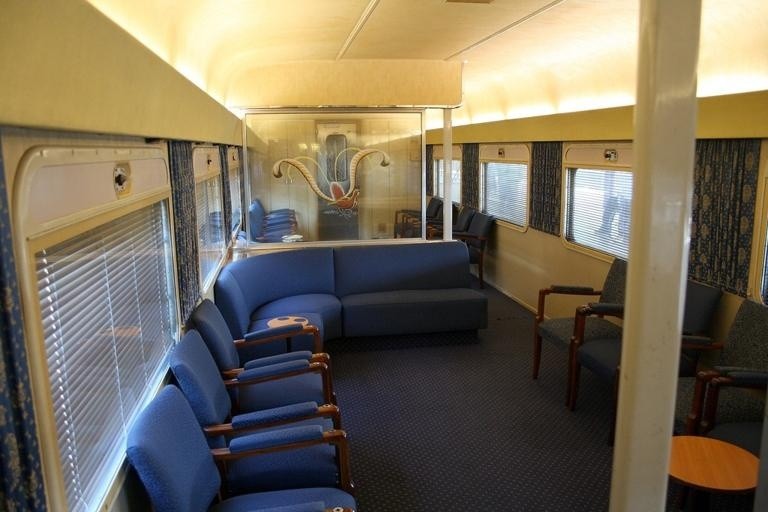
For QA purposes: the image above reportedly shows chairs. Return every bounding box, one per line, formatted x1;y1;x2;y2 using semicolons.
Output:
190;297;338;414
392;196;497;288
531;257;768;454
123;385;358;512
169;329;342;468
247;198;300;240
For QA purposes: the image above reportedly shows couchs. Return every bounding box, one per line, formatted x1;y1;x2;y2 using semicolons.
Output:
211;235;489;355
